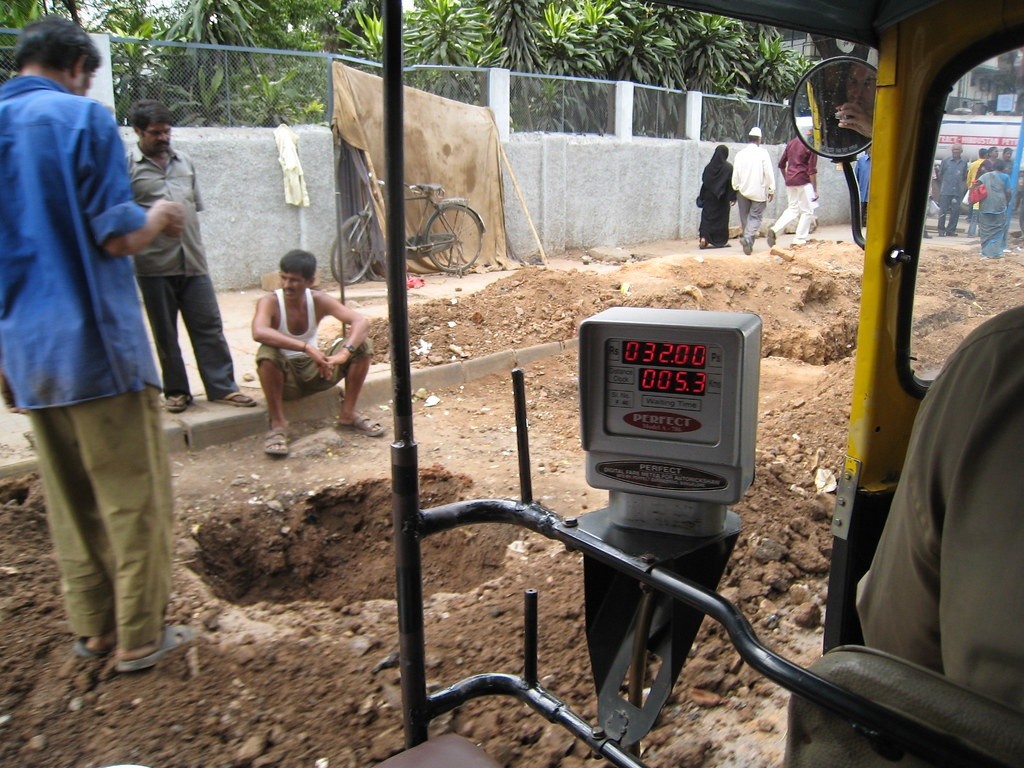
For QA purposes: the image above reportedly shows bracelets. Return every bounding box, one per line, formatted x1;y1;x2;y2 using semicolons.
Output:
303;343;307;352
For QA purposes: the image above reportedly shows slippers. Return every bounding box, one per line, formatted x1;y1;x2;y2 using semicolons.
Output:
260;426;291;452
337;414;388;439
211;390;254;408
160;390;187;414
117;627;203;673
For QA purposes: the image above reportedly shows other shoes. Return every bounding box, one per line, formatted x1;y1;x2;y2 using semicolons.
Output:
939;232;958;236
738;236;755;255
767;230;776;248
923;233;932;238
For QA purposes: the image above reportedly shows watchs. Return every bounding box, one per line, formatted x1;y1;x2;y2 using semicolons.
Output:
342;343;358;355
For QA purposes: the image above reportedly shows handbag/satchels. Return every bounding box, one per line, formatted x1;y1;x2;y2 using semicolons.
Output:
967;179;988;204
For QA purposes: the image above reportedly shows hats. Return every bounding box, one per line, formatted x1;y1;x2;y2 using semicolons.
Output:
747;125;763;137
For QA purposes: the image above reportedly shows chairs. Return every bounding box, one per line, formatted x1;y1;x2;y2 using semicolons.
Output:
783;644;1024;768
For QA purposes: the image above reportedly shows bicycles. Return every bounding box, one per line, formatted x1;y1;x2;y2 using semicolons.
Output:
330;172;487;286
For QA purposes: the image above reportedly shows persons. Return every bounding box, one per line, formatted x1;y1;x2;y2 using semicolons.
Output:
0;14;199;674
122;99;257;411
922;143;1024;257
252;249;385;455
731;127;776;255
833;65;877;141
855;145;871;228
855;308;1023;715
698;145;736;249
767;129;819;248
